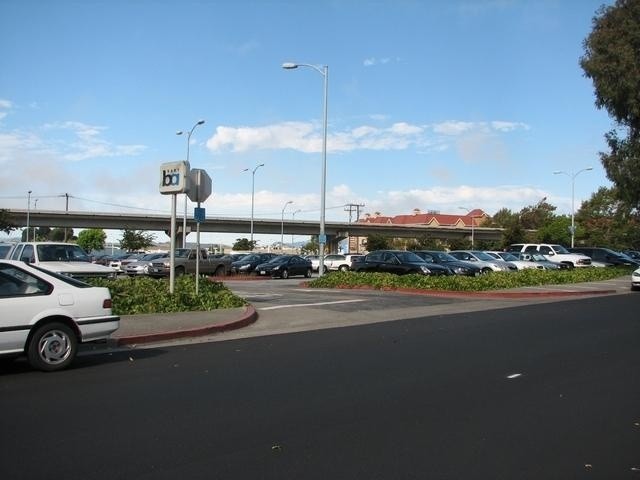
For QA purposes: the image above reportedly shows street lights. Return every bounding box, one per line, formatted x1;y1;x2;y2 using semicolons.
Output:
280;199;295;246
458;206;476;250
240;161;265;245
281;61;332;276
33;198;40;243
26;189;32;243
552;166;594;247
175;119;205;248
291;209;302;245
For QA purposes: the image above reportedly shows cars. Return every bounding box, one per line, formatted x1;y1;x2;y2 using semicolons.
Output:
0;258;122;374
631;264;639;290
